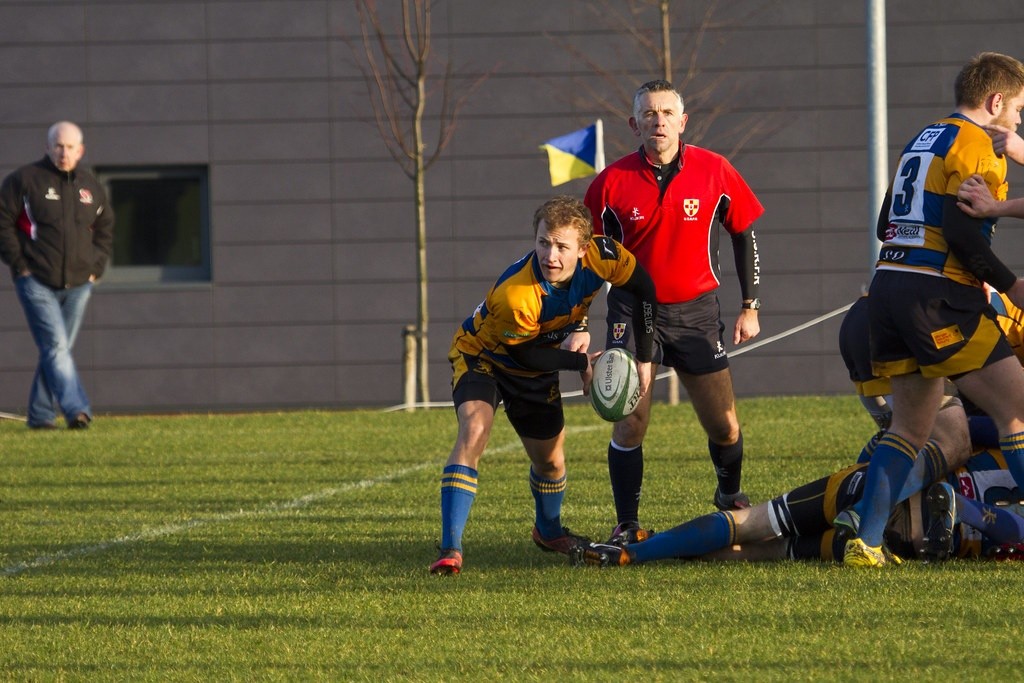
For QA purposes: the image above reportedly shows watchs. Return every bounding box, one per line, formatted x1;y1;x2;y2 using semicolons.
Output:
741;297;761;310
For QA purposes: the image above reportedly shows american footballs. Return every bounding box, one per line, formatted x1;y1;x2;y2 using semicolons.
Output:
589;345;643;421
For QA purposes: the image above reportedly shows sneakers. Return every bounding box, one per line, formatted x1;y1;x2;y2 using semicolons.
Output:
607;527;651;552
531;522;595;554
922;482;956;564
833;508;891;554
843;537;902;568
986;541;1024;563
612;522;641;539
567;541;622;568
713;487;752;512
430;548;463;575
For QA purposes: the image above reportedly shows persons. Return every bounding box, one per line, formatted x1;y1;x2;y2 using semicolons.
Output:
0;121;116;428
429;196;662;577
957;124;1024;221
585;78;766;549
844;52;1024;568
584;416;1024;568
832;280;1024;565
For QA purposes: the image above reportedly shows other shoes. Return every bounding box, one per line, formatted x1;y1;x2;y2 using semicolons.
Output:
71;414;88;429
31;421;59;431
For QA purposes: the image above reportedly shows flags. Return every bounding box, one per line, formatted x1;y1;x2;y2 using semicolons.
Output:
540;121;605;186
